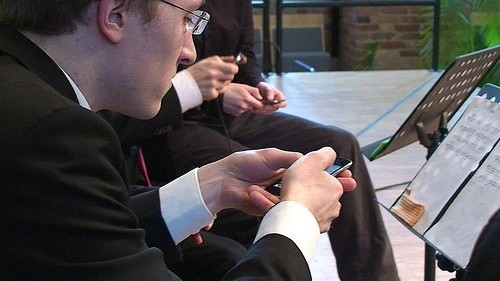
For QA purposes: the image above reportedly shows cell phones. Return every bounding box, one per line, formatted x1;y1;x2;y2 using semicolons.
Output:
267;156;353;197
231;52;243;64
257;99;278;105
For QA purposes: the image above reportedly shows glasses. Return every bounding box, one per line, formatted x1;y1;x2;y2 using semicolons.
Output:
160;0;210;35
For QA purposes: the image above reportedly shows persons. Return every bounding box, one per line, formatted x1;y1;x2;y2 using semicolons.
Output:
143;0;399;281
0;0;357;281
96;55;282;281
